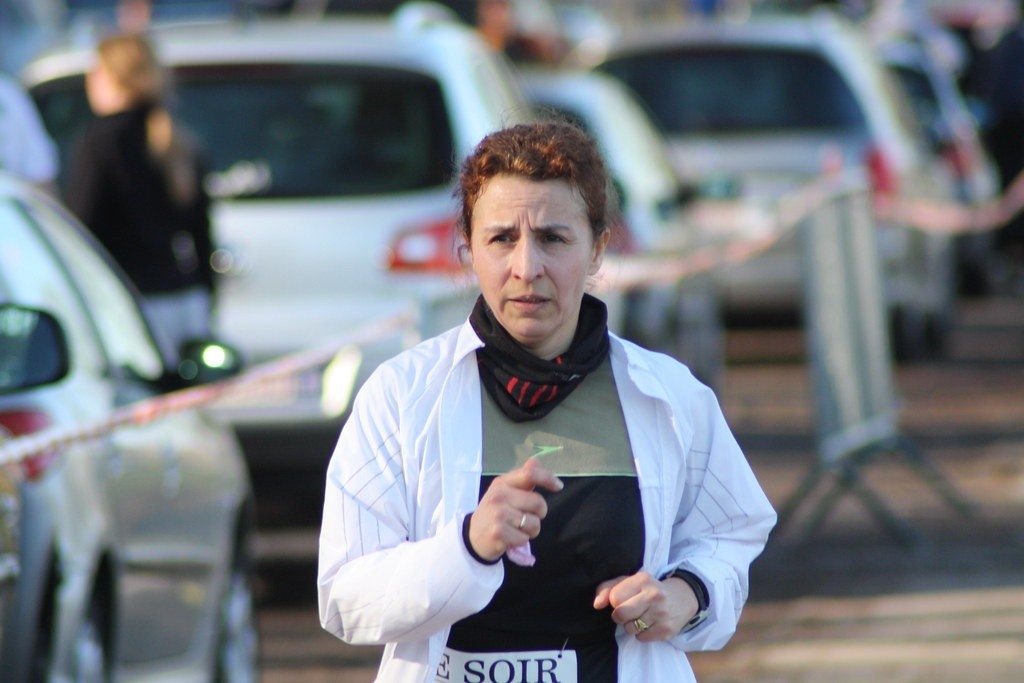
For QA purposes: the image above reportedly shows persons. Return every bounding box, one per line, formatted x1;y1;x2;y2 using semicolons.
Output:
34;34;216;356
317;118;777;683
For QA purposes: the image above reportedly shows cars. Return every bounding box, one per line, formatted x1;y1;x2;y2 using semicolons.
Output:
588;1;1004;369
0;172;274;683
516;55;749;407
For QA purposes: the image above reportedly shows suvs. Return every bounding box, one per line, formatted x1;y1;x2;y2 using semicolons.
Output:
13;0;631;514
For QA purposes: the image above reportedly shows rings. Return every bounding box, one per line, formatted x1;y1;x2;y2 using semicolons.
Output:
519;513;526;530
635;619;649;634
